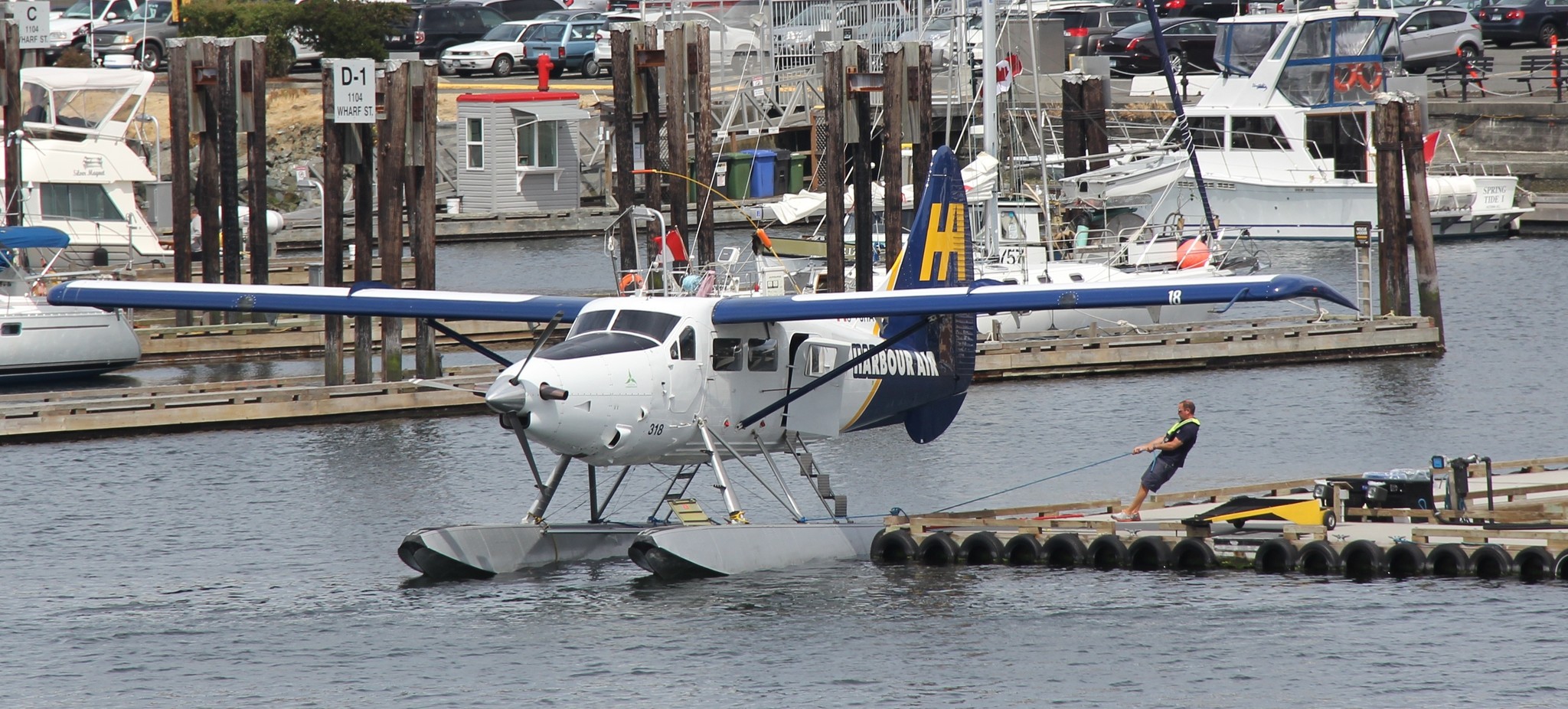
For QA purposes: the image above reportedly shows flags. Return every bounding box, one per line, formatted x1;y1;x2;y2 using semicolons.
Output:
652;230;688;264
996;54;1023;96
1421;130;1440;164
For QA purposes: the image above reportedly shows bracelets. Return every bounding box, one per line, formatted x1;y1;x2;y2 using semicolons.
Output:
1153;445;1156;449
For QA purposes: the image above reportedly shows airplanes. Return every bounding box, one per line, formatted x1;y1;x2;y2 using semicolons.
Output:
48;146;1366;582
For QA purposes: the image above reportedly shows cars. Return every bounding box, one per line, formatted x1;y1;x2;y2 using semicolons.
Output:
379;0;612;77
279;24;337;73
1480;0;1568;49
594;0;799;77
43;0;188;71
593;0;1496;82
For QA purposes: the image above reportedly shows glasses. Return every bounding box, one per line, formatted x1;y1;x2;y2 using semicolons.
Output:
1178;409;1186;413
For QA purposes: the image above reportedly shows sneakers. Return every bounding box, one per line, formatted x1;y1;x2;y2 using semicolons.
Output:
1131;513;1140;521
1111;510;1132;522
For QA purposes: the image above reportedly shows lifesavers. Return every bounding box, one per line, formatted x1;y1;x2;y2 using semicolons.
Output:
1425;544;1468;570
958;531;1004;562
1468;544;1513;571
1296;540;1339;566
1002;533;1041;560
1384;542;1426;570
1339;539;1383;566
38;271;61;297
1255;538;1296;562
1357;62;1382;90
1039;533;1088;560
870;527;918;560
619;273;646;297
1513;547;1554;572
1127;536;1172;563
1552;549;1568;574
1171;538;1215;563
915;532;959;563
1086;534;1127;561
1335;64;1356;92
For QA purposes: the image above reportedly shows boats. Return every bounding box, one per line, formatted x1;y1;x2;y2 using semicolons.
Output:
989;9;1536;268
0;65;287;382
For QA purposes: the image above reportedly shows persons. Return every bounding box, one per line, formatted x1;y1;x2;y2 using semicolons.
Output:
1111;400;1200;521
189;206;203;261
